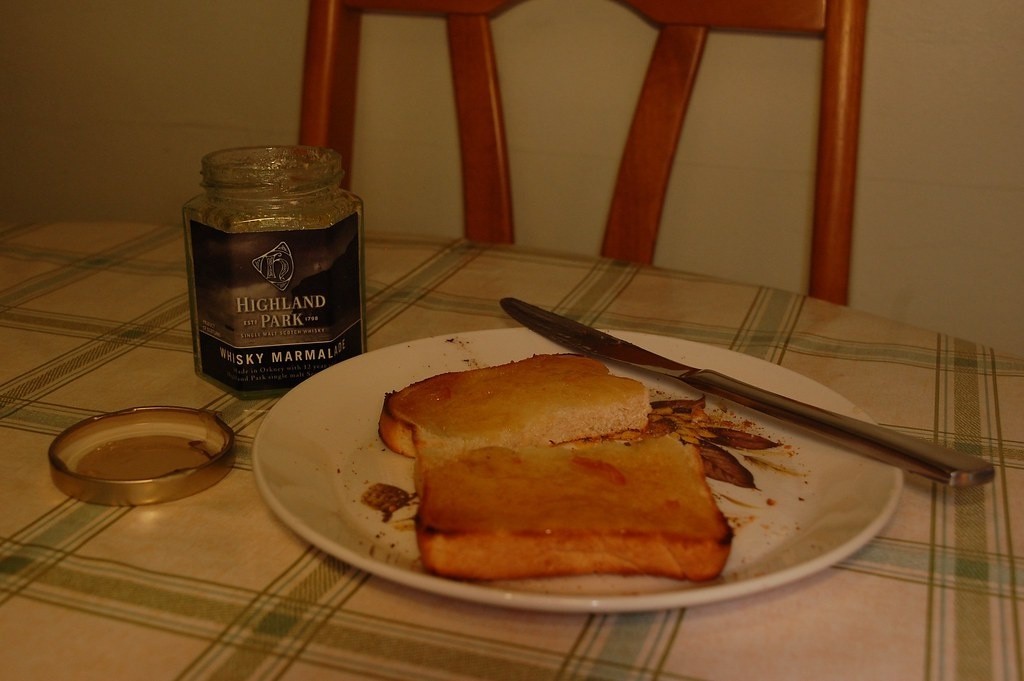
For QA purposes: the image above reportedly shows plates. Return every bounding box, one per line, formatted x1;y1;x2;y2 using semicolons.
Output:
252;326;903;612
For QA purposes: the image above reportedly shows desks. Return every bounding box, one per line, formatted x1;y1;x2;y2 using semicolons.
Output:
0;219;1024;681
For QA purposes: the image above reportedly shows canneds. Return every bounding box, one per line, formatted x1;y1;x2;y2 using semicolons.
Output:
182;144;367;399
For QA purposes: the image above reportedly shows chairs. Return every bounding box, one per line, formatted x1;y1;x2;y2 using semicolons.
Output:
295;0;874;304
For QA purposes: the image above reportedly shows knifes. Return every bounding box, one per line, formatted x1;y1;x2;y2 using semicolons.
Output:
502;296;996;489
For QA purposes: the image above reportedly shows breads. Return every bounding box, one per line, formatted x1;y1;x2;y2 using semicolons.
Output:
377;354;734;582
